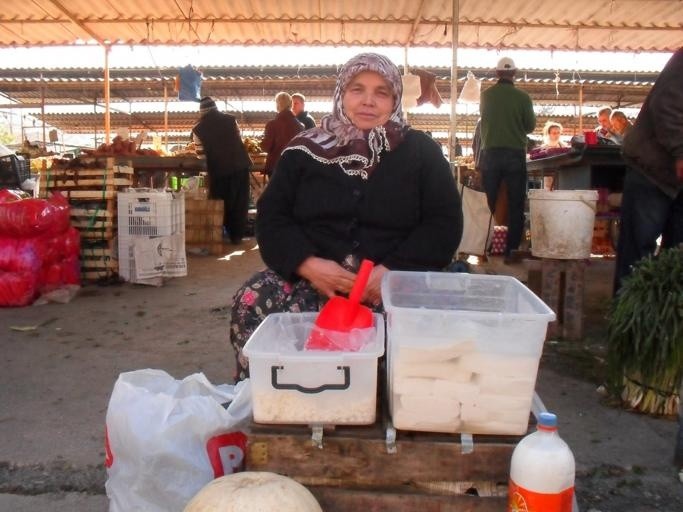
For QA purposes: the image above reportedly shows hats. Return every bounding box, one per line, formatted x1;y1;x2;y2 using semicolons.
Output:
200;96;217;114
496;57;516;70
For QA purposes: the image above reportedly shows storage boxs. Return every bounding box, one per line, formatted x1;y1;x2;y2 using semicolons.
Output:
381;271;557;437
242;311;385;435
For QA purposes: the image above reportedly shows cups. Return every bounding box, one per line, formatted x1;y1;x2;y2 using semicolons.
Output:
584;131;598;145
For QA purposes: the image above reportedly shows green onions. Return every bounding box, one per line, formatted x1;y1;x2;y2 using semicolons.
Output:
600;246;683;420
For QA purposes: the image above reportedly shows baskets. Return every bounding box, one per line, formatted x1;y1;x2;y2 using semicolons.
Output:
0;153;31;186
117;186;186;288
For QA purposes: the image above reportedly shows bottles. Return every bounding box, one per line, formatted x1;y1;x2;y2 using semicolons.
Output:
506;411;576;512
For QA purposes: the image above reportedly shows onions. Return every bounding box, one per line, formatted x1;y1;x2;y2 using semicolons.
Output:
491;226;509;253
529;148;546;159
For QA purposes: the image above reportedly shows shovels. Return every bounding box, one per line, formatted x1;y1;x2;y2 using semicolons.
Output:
305;260;374;351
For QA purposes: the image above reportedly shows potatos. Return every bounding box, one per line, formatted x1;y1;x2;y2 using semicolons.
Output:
456;154;474;165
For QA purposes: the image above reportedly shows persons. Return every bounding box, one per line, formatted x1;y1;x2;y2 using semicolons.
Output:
594;107;635;144
290;93;318;131
611;47;682;299
260;92;305;182
191;95;254;245
472;56;537;263
540;120;568;149
229;54;464;387
